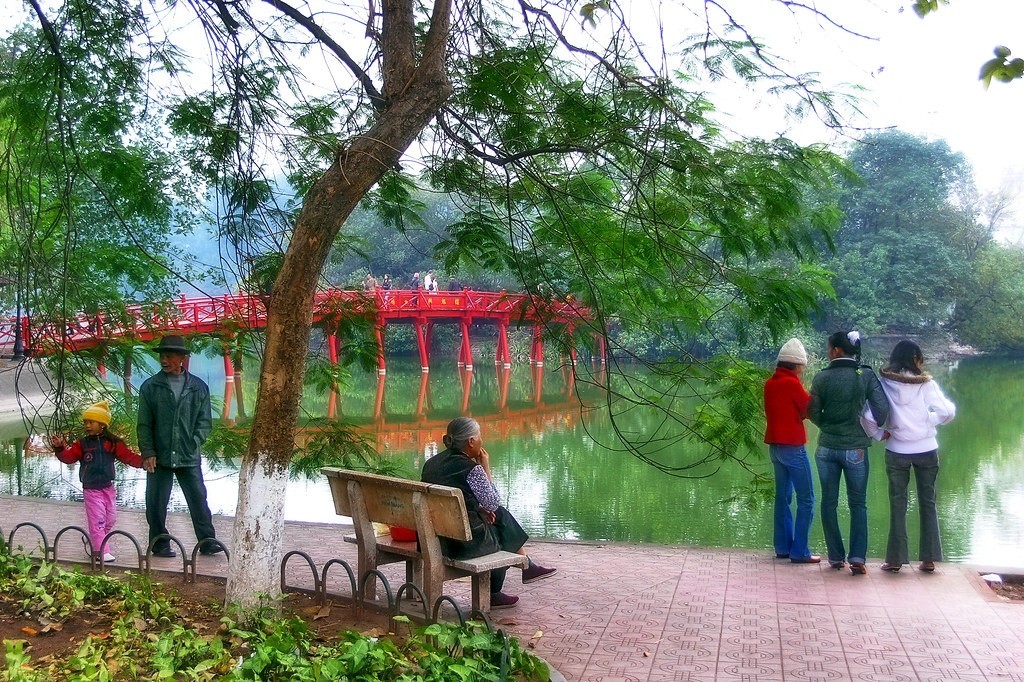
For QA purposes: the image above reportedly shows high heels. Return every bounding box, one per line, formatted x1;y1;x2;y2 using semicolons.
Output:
849;562;867;576
919;561;934;573
832;563;844;570
881;562;902;573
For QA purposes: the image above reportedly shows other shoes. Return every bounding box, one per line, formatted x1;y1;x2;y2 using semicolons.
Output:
202;543;224;555
154;547;176;557
776;553;789;558
791;556;820;563
95;553;115;562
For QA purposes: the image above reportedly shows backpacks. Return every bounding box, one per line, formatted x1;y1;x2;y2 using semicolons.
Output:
429;283;434;290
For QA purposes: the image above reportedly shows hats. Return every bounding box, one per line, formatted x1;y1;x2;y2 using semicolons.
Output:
449;275;455;278
152;335;191;354
779;338;807;365
82;401;111;429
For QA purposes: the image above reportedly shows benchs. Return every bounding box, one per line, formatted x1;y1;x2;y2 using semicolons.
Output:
320;467;528;621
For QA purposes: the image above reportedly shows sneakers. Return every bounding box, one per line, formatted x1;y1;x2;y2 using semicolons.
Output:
522;563;558;584
490;592;520;609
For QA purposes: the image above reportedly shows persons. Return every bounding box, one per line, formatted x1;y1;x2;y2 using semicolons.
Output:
859;340;956;574
763;339;822;562
52;399;154;563
808;331;889;575
358;272;464;305
137;335;224;556
415;417;558;610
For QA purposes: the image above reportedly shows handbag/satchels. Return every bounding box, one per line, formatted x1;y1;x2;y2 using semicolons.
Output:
388;525;416;541
